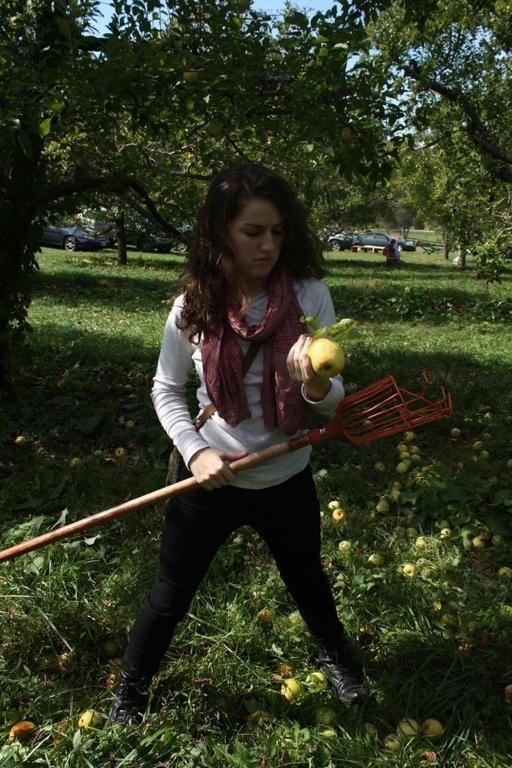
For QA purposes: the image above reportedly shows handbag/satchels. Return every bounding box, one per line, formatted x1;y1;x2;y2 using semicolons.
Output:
163;446;187;487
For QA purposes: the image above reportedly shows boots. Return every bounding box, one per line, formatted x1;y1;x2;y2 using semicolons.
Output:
105;668;150;733
317;644;367;704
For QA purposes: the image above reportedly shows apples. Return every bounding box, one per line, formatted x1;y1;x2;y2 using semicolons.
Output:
308;336;344;377
8;371;512;763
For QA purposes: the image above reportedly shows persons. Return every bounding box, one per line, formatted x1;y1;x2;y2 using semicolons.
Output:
114;159;378;727
382;239;401;267
